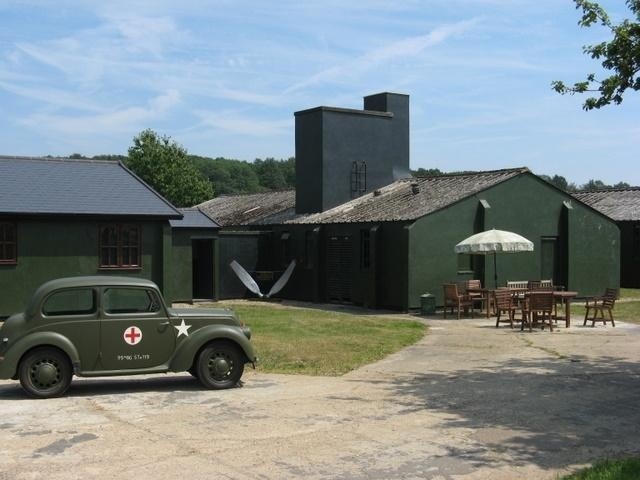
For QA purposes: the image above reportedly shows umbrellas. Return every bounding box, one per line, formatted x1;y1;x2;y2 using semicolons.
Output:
453;227;535;290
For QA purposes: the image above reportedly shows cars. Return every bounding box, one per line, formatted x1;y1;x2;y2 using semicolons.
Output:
0;274;258;398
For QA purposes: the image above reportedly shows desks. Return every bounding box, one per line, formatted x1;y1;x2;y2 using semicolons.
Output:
523;290;579;329
465;285;492;319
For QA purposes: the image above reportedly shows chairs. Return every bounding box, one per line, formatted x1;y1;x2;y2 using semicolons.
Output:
536;280;558;323
583;287;619;328
522;290;554;334
493;288;522;329
465;279;485;314
443;283;474;320
507;281;529;321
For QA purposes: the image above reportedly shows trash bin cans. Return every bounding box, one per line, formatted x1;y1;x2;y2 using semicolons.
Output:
420;294;436;316
